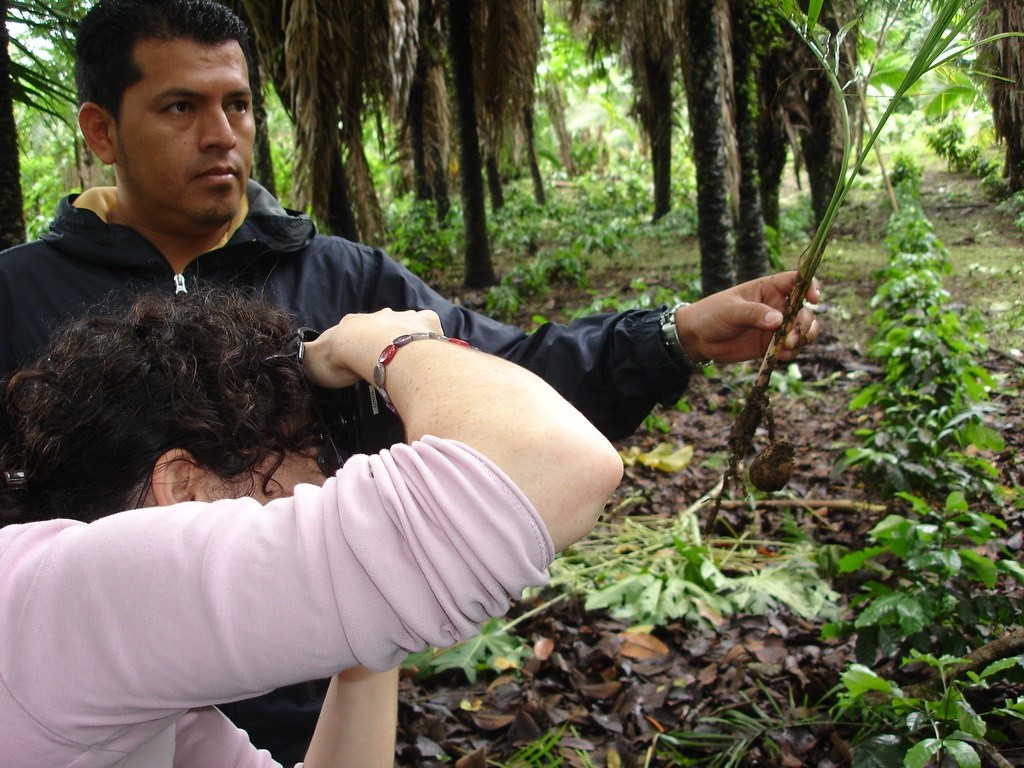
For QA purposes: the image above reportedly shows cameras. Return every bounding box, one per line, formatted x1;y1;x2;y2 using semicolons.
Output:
330;379;407;459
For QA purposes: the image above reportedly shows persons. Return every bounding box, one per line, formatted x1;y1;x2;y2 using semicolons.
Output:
0;296;624;768
0;0;819;768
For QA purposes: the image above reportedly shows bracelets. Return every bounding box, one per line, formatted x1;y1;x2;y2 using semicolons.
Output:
659;303;714;368
374;332;479;416
